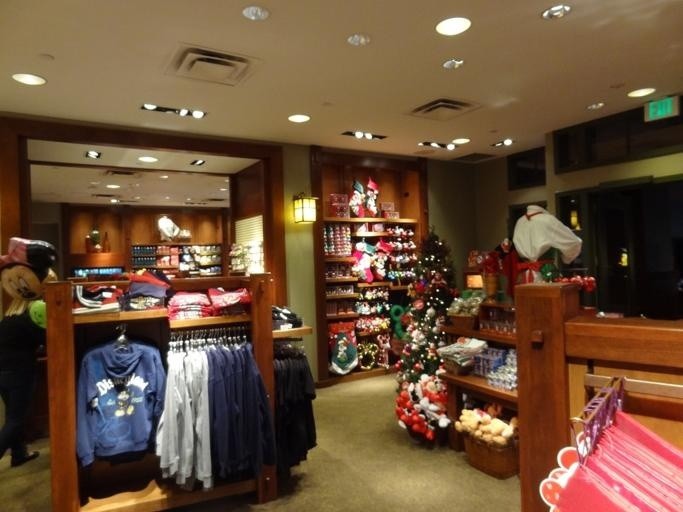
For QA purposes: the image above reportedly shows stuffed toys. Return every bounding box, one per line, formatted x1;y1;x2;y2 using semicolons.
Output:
394;374;520;448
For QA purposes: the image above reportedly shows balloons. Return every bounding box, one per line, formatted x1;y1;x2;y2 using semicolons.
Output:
0;236;59;301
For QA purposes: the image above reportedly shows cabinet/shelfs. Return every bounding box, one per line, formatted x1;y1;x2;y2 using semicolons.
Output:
310;145;428;390
42;271;312;512
437;298;518;454
126;242;222;276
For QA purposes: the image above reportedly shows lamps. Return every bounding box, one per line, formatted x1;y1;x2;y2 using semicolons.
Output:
291;192;318;227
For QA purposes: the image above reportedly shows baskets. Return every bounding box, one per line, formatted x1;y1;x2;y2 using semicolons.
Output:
443;358;473;376
460;430;519;480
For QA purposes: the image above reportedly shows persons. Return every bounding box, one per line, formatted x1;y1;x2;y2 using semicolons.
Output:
0;298;49;468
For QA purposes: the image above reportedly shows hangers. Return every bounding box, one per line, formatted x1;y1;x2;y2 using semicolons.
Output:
104;322;308;364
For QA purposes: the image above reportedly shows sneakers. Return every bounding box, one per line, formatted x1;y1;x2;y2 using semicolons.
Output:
11;451;39;467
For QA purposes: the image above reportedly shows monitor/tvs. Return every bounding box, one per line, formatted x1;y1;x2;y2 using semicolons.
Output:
73;265;125;277
